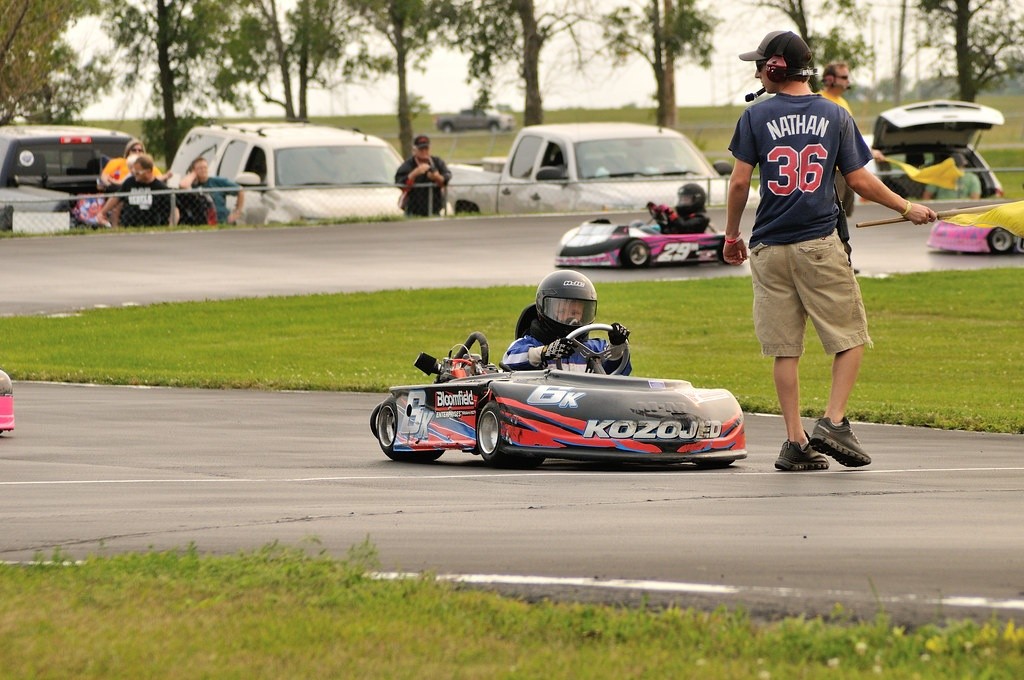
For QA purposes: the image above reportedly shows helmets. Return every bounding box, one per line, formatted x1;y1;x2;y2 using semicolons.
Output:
535;270;598;338
674;183;706;216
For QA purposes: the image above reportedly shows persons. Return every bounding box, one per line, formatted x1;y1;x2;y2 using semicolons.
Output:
723;30;938;470
93;140;171;227
502;270;632;376
630;183;710;234
396;135;453;217
923;153;982;199
817;62;885;217
180;157;245;225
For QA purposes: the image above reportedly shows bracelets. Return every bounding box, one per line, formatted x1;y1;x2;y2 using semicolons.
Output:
902;201;911;216
726;232;742;243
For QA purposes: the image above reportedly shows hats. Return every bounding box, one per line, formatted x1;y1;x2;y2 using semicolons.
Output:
739;30;811;71
414;135;430;148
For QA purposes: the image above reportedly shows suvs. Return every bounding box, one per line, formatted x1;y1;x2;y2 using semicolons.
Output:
164;121;407;226
0;124;145;226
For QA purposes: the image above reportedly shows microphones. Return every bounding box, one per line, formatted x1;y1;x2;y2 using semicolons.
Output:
745;88;765;102
847;86;851;89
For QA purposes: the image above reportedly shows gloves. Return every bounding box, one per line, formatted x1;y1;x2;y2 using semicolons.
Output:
541;338;578;362
608;323;630;346
655;204;672;217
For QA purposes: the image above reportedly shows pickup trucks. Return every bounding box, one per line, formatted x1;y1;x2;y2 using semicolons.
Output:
440;119;757;216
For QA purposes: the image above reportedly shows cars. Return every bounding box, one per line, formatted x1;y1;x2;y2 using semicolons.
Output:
854;99;1006;209
437;106;517;134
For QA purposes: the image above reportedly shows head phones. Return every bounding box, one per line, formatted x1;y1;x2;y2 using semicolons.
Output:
766;31;794;82
822;65;836;87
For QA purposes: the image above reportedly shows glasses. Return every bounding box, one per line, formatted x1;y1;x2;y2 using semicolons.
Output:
756;60;768;72
833;75;848;80
130;149;143;152
132;169;149;177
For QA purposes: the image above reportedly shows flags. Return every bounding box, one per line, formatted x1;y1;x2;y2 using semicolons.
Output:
940;200;1024;239
888;157;963;191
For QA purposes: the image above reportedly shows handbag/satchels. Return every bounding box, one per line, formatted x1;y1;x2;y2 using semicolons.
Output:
398;190;408;210
646;202;658;211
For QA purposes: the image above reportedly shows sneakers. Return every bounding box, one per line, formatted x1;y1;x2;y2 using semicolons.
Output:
809;416;872;468
774;430;830;471
